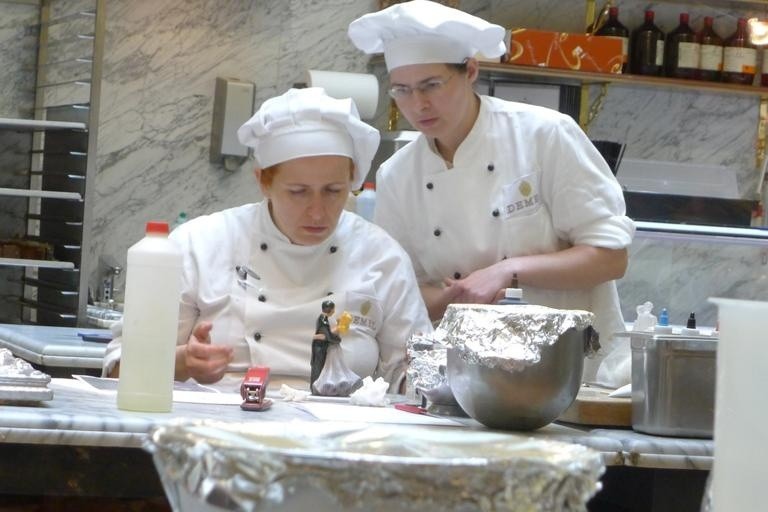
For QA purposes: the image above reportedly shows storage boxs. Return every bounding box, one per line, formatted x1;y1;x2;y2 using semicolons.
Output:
502;28;623;75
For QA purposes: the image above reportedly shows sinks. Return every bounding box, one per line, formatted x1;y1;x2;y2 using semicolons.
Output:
89;303;123;320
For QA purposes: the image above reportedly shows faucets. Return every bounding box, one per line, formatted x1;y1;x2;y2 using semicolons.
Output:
98;266;122;308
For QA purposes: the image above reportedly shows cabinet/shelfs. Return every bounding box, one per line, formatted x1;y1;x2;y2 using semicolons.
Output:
369;53;768;238
0;1;106;330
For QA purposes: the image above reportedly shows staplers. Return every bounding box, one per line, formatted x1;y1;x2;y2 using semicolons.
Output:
239;366;274;412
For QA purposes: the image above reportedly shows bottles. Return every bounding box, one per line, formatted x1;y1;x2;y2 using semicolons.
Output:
666;13;701;78
594;8;630;72
632;10;666;75
723;20;757;84
700;16;723;81
117;223;179;412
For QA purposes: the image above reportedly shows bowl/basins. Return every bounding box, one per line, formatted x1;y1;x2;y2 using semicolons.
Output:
444;304;586;432
412;340;457;406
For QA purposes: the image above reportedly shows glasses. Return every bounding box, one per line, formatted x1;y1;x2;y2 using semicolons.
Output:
386;57;471;95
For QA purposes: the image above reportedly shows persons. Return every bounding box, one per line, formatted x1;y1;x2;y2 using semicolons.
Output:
100;88;457;395
347;0;635;398
310;301;364;397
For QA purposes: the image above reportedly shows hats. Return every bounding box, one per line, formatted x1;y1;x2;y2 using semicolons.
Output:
346;1;506;74
237;86;382;192
